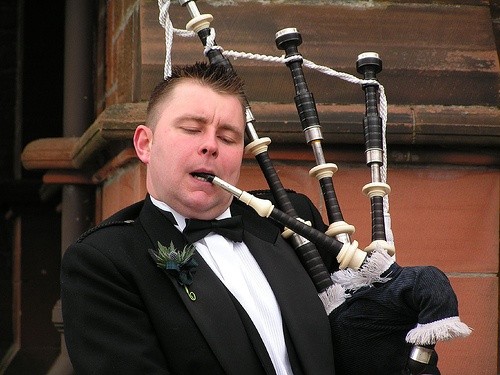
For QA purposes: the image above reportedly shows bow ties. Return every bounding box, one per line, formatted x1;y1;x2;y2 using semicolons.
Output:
180;212;248;244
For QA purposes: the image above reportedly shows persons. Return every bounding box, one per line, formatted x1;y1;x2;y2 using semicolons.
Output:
59;61;343;374
